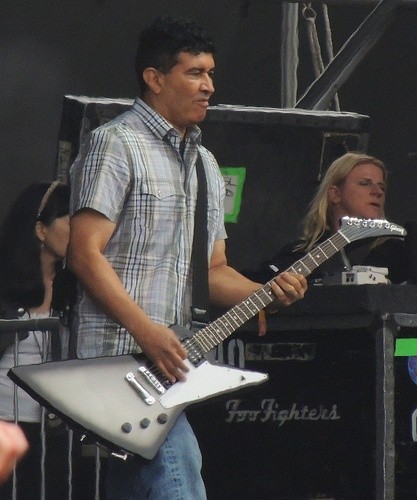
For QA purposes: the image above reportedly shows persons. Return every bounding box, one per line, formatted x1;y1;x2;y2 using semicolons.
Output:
60;20;309;500
278;151;413;286
0;180;77;493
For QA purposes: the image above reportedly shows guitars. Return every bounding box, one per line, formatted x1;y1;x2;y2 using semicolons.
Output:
7;215;408;466
